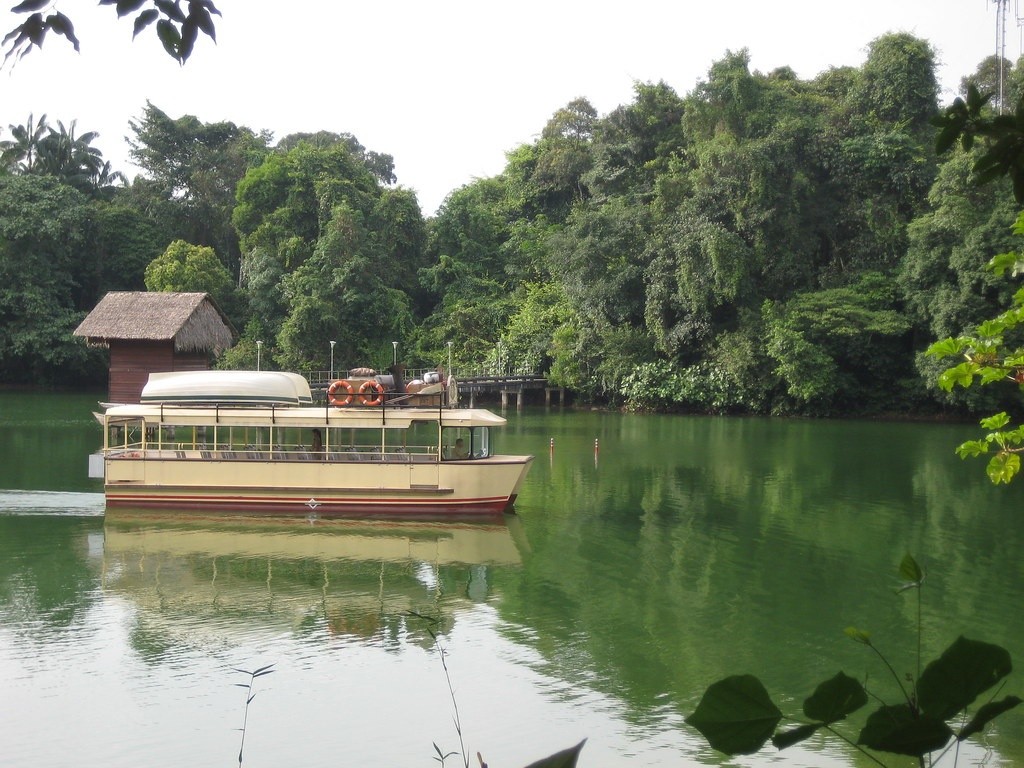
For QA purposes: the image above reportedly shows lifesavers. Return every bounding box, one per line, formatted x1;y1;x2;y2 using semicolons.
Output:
328;380;355;407
356;379;385;408
405;378;418;396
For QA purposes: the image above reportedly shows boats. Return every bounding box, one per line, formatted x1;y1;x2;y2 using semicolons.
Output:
102;369;537;517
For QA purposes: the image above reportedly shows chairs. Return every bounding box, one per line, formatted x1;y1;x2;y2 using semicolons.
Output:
369;446;388;460
320;446;341;461
198;444;212;459
269;445;288;459
219;444;238;459
345;447;364;460
243;443;264;459
395;446;408;461
294;446;314;460
173;445;187;458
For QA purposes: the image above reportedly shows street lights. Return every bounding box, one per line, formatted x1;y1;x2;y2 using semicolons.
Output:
392;340;399;365
330;340;337;379
256;340;264;371
447;341;454;376
497;342;502;377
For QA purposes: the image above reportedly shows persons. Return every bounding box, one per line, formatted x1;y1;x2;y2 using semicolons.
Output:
311;429;322;459
451;438;466;460
